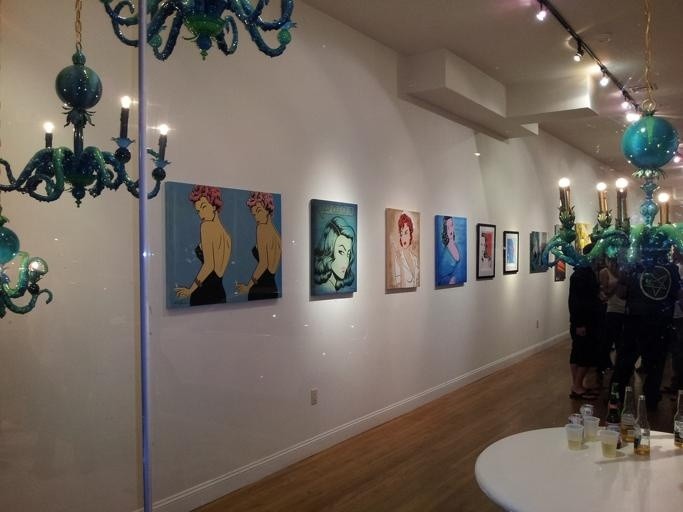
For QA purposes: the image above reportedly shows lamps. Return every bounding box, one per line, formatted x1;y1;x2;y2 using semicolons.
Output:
534;1;683;274
536;0;683;169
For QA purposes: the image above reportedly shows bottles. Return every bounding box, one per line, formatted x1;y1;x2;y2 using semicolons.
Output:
621;386;635;442
634;395;650;457
605;383;622;448
673;390;683;449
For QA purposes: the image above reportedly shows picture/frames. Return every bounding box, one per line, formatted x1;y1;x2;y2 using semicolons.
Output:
475;221;496;280
501;230;520;274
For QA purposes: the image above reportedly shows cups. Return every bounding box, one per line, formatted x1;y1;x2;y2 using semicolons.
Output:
564;424;584;450
596;430;620;457
584;417;600;441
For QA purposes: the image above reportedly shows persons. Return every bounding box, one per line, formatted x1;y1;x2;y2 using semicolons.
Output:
173;185;232;307
568;243;607;400
598;256;630;387
480;232;491;276
234;191;281;300
312;216;356;295
392;212;420;287
438;216;460;285
661;245;683;393
608;233;683;410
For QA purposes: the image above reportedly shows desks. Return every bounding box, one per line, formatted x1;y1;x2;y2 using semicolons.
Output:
473;425;683;512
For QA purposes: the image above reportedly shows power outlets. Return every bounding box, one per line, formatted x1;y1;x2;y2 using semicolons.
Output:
311;389;318;405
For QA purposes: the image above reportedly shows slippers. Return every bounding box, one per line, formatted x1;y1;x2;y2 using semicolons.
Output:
568;389;597;400
